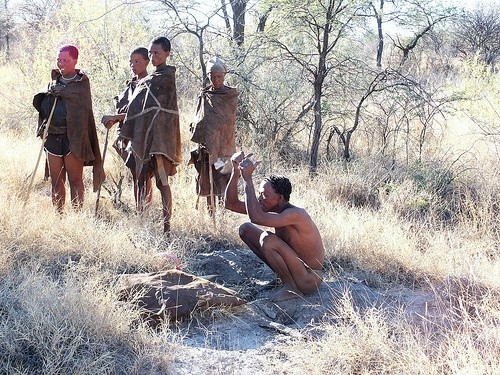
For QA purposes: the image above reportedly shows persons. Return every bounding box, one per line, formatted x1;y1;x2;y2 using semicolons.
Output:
101;47;153;215
222;151;325;303
188;56;239;216
119;36;181;246
33;45;105;220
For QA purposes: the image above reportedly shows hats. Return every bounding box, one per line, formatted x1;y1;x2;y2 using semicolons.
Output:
206;56;227;74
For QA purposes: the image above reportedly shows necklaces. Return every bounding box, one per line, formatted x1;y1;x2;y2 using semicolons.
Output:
62;74;77;80
